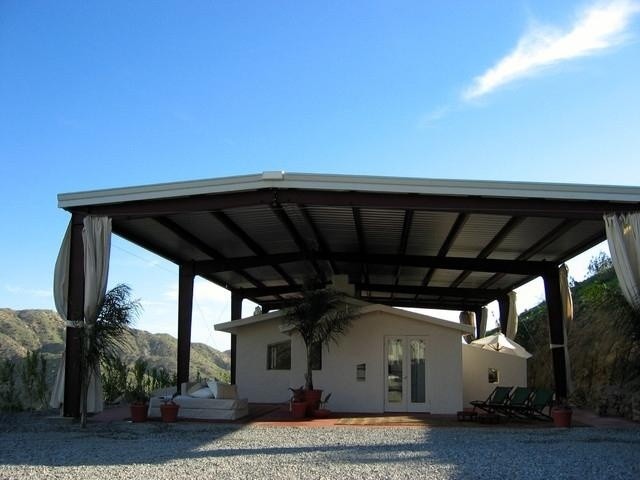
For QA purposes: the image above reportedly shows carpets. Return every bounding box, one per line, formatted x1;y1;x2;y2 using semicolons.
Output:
333;413;462;427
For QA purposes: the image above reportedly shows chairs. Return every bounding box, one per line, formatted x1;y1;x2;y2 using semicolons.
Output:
469;384;558;425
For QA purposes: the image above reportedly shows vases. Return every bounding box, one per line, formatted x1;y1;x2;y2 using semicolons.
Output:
550;396;579;427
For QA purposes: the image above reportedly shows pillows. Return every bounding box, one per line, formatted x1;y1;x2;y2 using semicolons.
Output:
207;377;236;399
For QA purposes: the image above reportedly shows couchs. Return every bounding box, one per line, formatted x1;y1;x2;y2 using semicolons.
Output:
147;381;248;420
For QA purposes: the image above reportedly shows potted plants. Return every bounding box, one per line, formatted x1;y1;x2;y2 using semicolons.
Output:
278;288;366;418
128;358;178;422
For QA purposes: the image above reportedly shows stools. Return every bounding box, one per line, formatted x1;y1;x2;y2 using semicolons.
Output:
457;411;478;422
